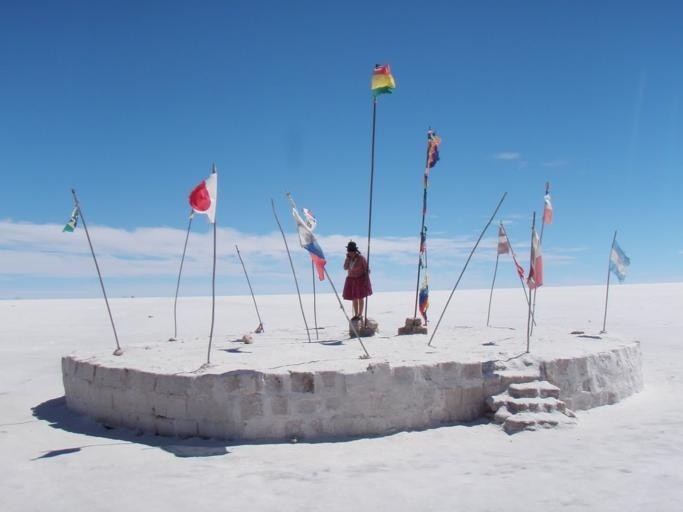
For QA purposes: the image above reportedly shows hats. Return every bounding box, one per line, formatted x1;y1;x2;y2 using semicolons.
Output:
346;242;358;250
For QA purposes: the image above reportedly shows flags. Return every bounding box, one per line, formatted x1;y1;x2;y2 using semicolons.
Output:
498;225;510;255
60;206;78;234
301;205;317;231
524;227;544;290
288;196;325;282
607;240;631;285
542;186;553;223
515;263;524;281
369;63;396;103
184;170;217;224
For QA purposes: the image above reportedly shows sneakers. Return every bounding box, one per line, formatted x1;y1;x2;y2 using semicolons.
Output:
351;315;361;320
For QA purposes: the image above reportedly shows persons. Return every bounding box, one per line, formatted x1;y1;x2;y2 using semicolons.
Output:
340;240;372;320
